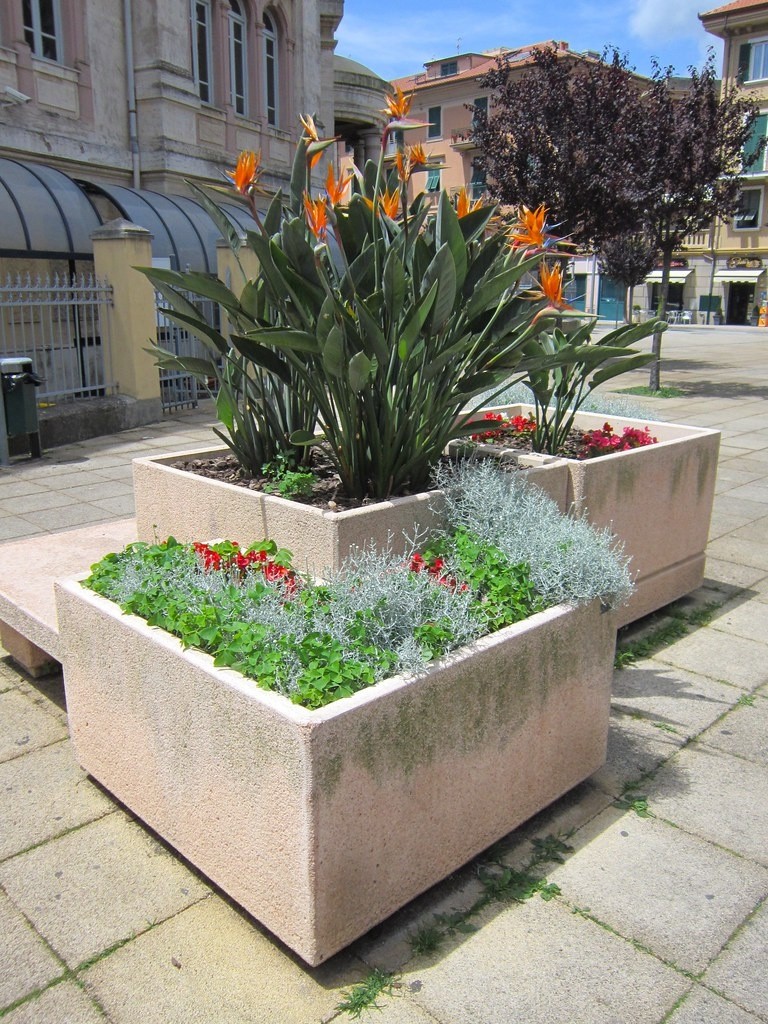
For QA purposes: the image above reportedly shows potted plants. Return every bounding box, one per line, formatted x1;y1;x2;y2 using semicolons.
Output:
713;305;723;325
632;305;641;313
751;305;759;326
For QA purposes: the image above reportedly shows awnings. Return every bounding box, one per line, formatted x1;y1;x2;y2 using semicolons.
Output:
0;155;299;275
713;268;766;284
644;268;694;284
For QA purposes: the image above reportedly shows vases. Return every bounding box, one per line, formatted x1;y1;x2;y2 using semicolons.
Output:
55;403;720;967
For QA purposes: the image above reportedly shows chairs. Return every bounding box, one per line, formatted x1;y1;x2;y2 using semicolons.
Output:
647;311;692;324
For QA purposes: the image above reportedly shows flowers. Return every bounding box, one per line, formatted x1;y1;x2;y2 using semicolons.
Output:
129;80;658;498
87;450;638;710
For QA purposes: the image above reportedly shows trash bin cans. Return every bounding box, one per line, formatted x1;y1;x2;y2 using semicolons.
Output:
0;356;42;458
